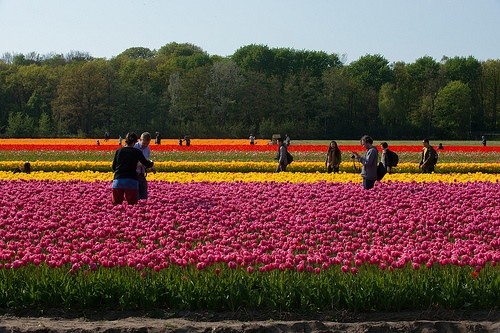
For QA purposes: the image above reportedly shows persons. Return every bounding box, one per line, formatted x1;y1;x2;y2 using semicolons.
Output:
325;141;342;173
275;138;289;172
178;138;183;146
133;131;155;200
285;134;290;147
118;135;122;145
481;136;486;146
438;144;444;150
355;135;379;189
155;131;161;145
96;141;100;146
418;139;438;173
248;134;255;144
110;131;154;205
13;162;31;174
185;136;191;146
104;131;109;142
379;142;392;174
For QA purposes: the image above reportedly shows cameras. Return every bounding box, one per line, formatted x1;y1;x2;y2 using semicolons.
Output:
350;151;357;159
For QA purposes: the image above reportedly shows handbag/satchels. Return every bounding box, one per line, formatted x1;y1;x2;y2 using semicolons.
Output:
376;162;388;181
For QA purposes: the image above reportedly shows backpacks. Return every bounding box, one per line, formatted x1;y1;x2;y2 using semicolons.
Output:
279;145;293;164
385;149;399;167
428;146;438;165
